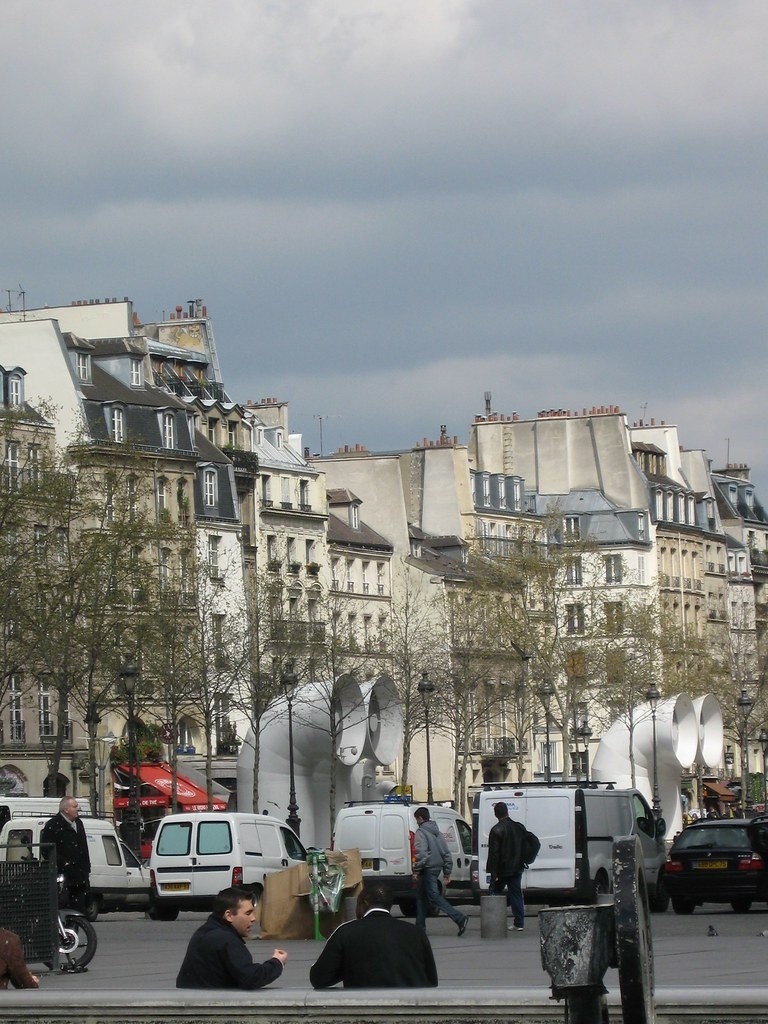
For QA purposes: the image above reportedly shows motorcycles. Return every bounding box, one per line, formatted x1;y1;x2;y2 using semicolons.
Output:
19;842;98;973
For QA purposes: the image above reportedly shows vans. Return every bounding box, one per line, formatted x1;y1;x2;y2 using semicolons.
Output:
468;781;670;914
0;793;150;922
148;812;309;922
330;800;475;917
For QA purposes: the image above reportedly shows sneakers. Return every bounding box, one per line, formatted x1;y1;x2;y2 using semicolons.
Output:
507;924;523;930
458;916;468;935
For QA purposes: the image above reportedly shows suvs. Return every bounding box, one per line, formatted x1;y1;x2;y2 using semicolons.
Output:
663;815;768;914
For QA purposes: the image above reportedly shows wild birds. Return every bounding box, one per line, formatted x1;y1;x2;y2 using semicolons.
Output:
511;640;537;660
708;925;718;937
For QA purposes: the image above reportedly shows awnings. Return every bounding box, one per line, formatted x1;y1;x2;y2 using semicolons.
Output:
119;763;227;812
703;782;735;802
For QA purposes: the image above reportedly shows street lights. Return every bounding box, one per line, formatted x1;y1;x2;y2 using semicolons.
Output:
758;728;768;809
645;682;662;820
534;678;557;782
737;690;752;810
118;648;142;869
580;720;594;781
416;671;436;805
279;660;302;839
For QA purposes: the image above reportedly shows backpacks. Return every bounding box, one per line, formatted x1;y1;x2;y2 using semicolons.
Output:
515;822;540;869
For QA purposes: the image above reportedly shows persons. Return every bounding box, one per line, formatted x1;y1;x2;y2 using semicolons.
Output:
310;885;439;988
683;811;698;824
0;928;39;990
40;796;91;933
176;887;288;989
486;802;525;930
707;807;720;818
730;804;741;818
412;807;469;936
